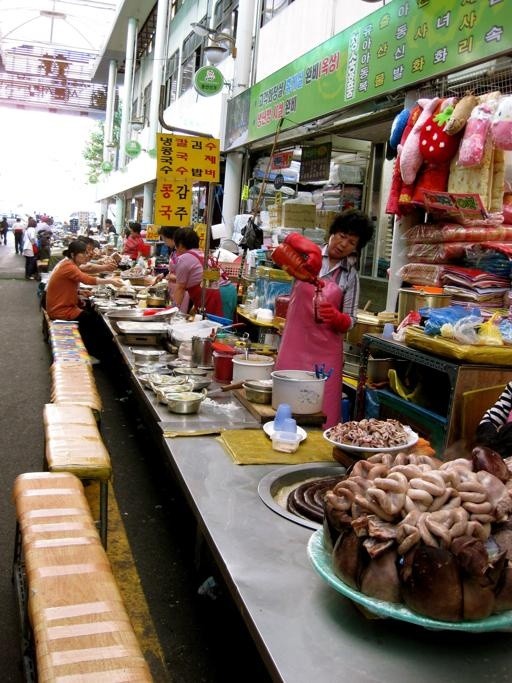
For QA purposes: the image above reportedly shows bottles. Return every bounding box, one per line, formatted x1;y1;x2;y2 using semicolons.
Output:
314;289;326;324
209;327;216;338
245;282;256;308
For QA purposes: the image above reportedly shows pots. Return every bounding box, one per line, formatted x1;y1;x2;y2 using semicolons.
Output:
159;389;208;413
397;286;453;322
131;348;167;368
156;383;194;405
147;373;190;387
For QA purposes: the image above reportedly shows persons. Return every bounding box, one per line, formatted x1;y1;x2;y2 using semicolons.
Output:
477;375;512;462
0;213;239;328
273;208;375;431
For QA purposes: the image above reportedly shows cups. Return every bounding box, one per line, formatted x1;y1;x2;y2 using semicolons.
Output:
281;417;298;440
383;324;395;340
273;404;292;432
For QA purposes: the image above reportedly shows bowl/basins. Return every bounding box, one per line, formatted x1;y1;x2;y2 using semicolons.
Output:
240;384;272;403
177;374;214;390
147;295;167;307
106;309;175;334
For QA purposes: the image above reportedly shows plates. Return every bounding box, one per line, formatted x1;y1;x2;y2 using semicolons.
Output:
306;520;511;637
137;369;172;379
135;361;165;370
323;420;419;454
263;420;307;444
243;378;273;390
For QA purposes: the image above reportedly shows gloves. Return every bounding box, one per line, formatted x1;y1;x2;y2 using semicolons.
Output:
319;302;351;332
285;232;323;276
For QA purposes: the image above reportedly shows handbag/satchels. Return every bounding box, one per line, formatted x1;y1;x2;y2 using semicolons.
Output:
33;244;38;253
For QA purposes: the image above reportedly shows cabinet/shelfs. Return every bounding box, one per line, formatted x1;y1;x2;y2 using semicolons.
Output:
353;333;512;460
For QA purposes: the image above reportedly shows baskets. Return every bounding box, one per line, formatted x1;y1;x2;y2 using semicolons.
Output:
219;262;249;278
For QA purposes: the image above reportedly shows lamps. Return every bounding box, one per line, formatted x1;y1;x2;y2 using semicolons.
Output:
189;24;236;66
130;116;149;131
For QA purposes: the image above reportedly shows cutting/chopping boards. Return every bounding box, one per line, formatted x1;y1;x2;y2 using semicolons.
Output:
233;387;326;424
